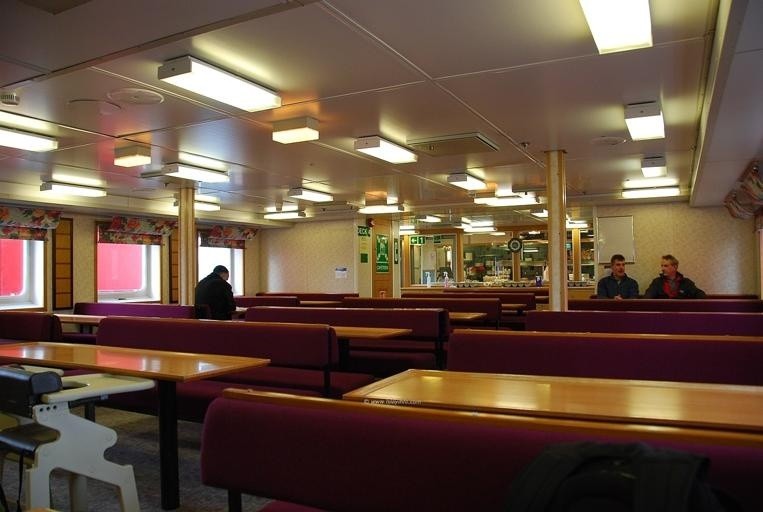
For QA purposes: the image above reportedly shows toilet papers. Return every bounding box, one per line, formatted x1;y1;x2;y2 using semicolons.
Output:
335;267;347;279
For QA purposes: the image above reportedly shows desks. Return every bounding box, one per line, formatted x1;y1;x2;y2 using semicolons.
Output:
0;341;270;511
342;367;763;438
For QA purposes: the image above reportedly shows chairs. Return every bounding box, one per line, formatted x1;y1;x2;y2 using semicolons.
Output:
0;364;158;510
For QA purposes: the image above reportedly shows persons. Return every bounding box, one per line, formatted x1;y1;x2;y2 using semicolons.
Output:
598;253;639;301
640;254;706;296
194;264;235;320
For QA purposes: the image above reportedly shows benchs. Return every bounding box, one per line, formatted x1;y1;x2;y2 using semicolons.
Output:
201;388;762;511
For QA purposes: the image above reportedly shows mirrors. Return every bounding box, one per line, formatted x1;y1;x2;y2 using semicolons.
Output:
407;235;457;287
459;233;515;284
518;231;572;283
577;228;596;282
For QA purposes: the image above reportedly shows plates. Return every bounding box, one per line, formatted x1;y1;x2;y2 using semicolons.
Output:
507;237;522;253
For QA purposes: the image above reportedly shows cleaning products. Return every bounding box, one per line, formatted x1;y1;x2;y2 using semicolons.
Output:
444;272;449;288
425;272;431;288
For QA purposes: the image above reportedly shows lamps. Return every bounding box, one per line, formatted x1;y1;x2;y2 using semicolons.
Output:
38;182;107;199
0;125;58;153
622;100;665;142
159;164;229;184
577;0;653;56
173;198;220;213
111;146;151;168
156;57;281;113
269;117;320;146
